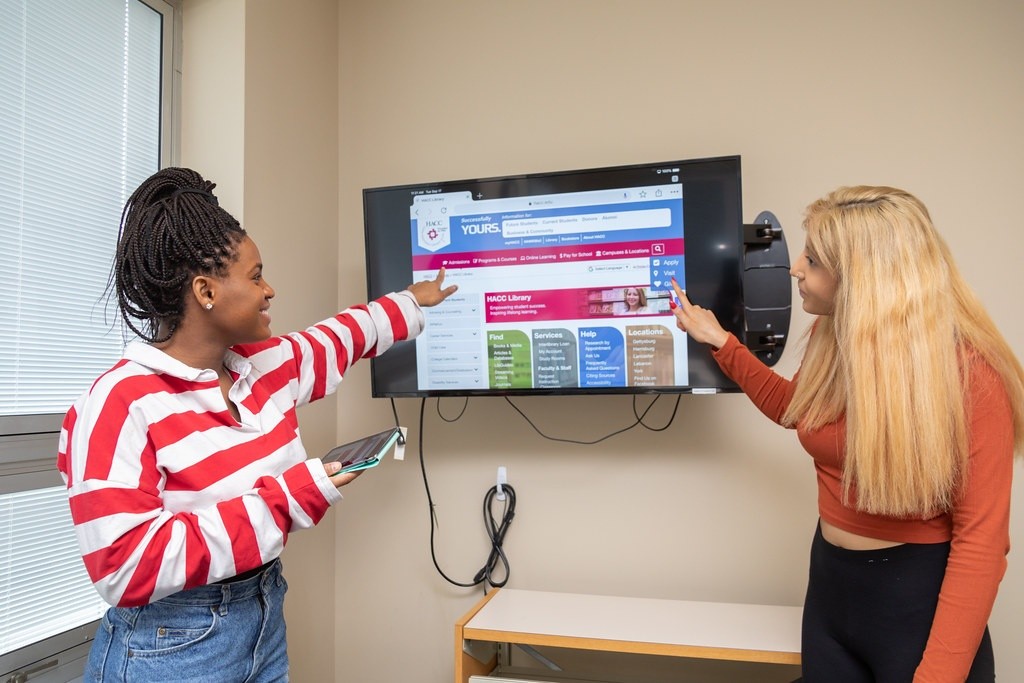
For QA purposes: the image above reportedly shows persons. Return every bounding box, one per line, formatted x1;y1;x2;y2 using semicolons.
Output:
57;168;458;683
624;289;648;313
670;184;1024;683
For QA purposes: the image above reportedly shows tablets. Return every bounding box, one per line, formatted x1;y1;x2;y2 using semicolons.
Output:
320;426;399;473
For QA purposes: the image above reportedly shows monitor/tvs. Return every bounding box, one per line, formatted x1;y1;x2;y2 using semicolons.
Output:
362;155;746;400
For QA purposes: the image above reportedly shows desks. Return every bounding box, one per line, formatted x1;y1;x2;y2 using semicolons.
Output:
453;587;803;683
